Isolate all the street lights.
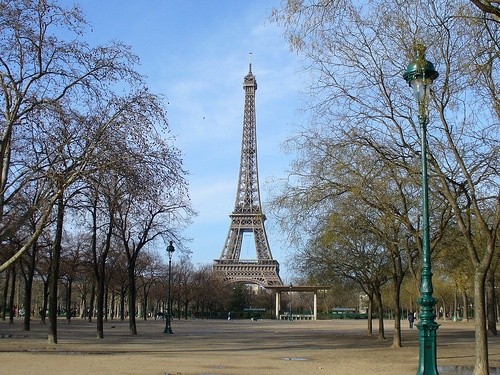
[289,284,293,321]
[163,241,175,334]
[402,43,442,375]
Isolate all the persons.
[408,311,414,328]
